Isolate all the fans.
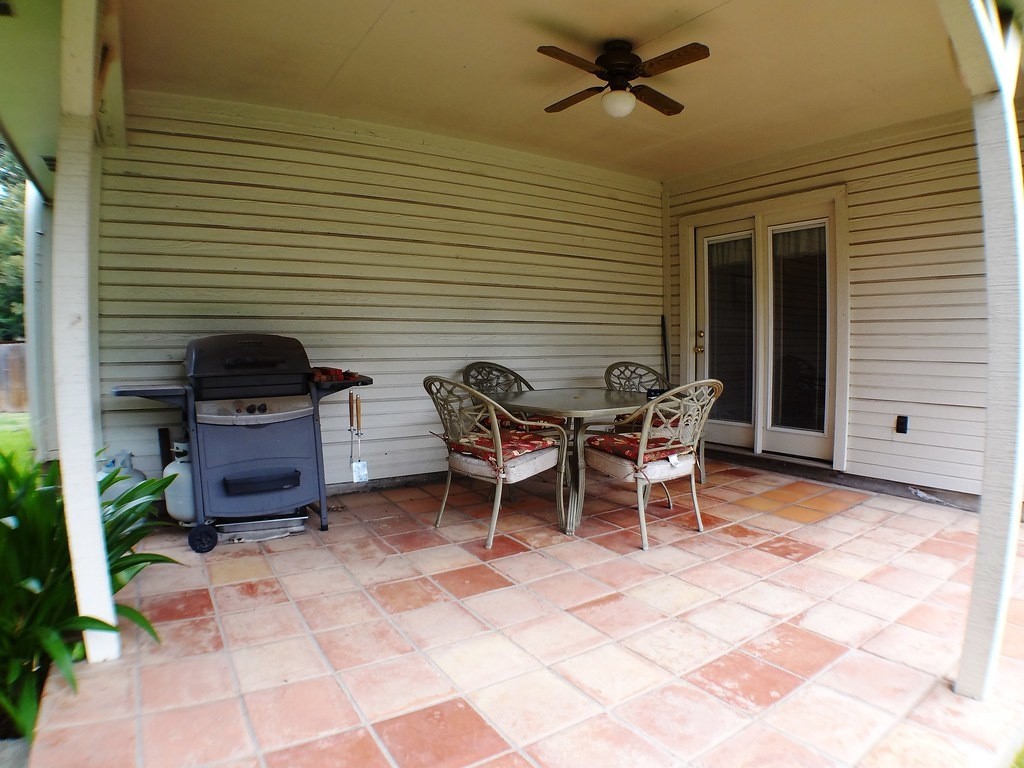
[536,39,710,117]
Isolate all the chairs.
[421,375,565,549]
[575,379,724,550]
[462,362,572,497]
[603,361,708,486]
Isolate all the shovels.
[352,394,368,483]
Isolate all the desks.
[476,387,688,536]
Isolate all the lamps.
[601,80,636,118]
